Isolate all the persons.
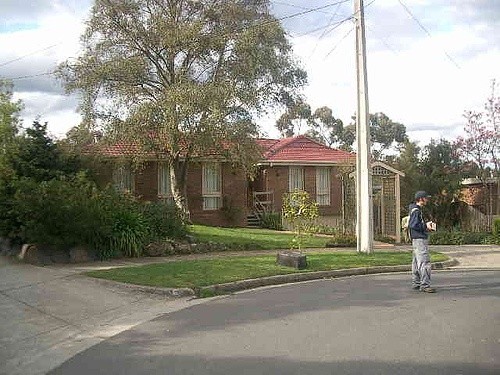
[408,191,436,293]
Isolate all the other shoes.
[421,287,436,292]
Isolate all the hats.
[414,191,431,200]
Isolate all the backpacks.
[402,208,423,243]
